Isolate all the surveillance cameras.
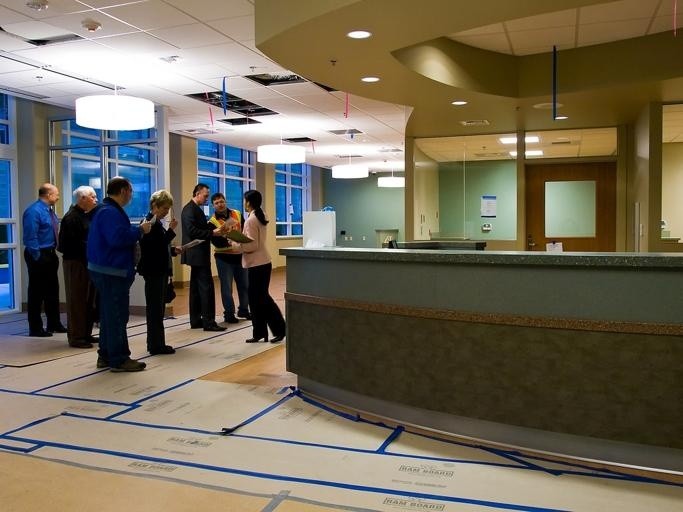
[27,0,46,11]
[82,22,99,30]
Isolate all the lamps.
[331,155,369,179]
[75,86,155,131]
[378,169,405,188]
[258,136,306,164]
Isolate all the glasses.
[166,206,176,223]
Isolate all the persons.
[241,190,287,343]
[88,177,152,373]
[137,190,184,356]
[22,182,67,336]
[208,192,251,324]
[58,186,99,351]
[181,182,233,332]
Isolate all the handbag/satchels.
[166,274,176,304]
[302,211,336,249]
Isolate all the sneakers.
[96,354,107,369]
[110,358,146,373]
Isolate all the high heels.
[270,332,287,343]
[247,333,269,343]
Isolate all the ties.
[49,206,60,250]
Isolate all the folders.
[227,230,254,243]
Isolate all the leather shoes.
[47,323,69,334]
[236,308,253,321]
[86,335,101,343]
[203,325,228,332]
[146,346,169,351]
[191,323,203,328]
[28,329,53,338]
[69,340,93,350]
[150,347,176,355]
[224,316,239,324]
